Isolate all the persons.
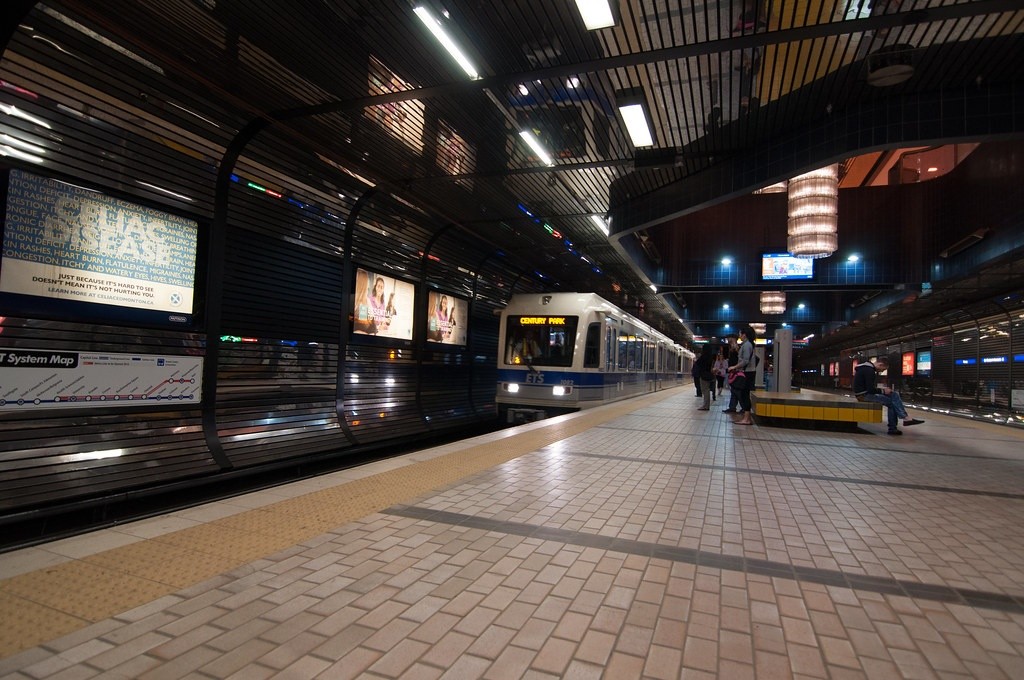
[429,291,456,342]
[691,325,755,424]
[793,369,801,386]
[853,357,925,435]
[355,270,396,334]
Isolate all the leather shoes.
[901,417,925,427]
[887,429,903,436]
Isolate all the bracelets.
[735,366,737,370]
[883,389,885,395]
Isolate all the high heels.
[718,390,722,396]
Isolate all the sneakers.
[722,408,737,414]
[735,408,746,414]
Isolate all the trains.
[491,292,697,426]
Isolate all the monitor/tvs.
[759,252,817,281]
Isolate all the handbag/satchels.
[728,369,747,390]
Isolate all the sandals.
[733,421,751,425]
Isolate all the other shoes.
[697,407,710,411]
[712,398,716,401]
[694,395,702,397]
